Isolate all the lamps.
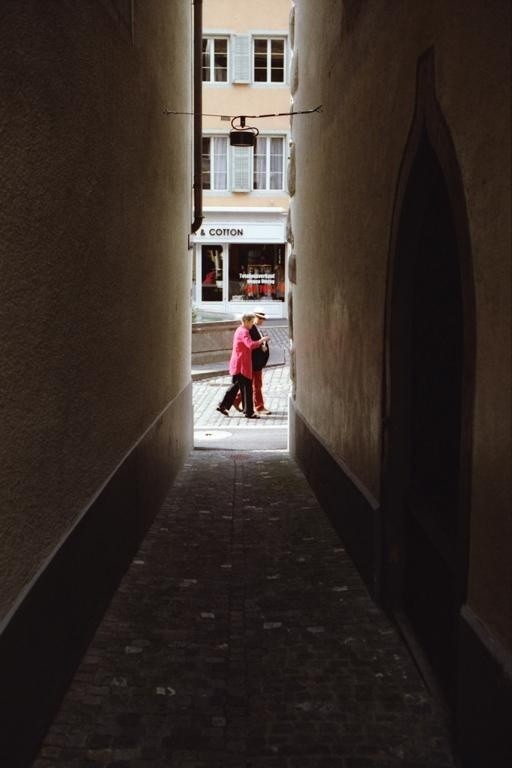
[229,117,259,147]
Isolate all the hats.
[253,310,266,319]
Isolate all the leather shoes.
[232,401,242,412]
[254,407,271,414]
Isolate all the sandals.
[216,405,228,416]
[245,413,260,419]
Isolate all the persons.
[216,313,271,419]
[233,312,272,414]
